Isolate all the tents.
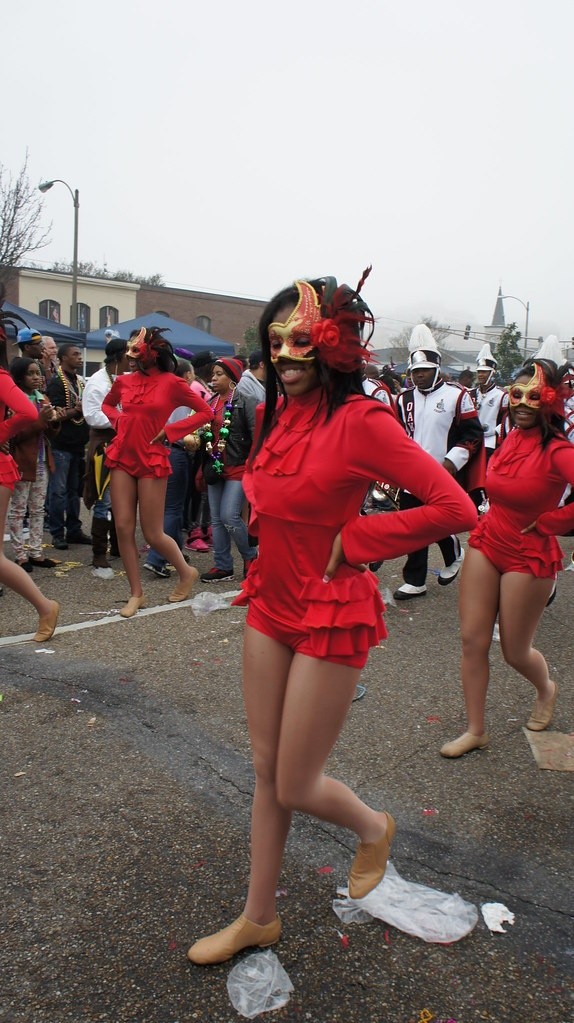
[1,301,87,379]
[85,313,235,357]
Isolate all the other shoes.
[169,567,200,602]
[120,591,145,617]
[33,600,59,642]
[28,556,56,568]
[347,811,395,899]
[369,560,384,572]
[166,553,190,566]
[438,548,465,586]
[16,560,33,572]
[91,566,114,580]
[526,680,558,731]
[109,547,121,557]
[142,562,171,576]
[439,732,490,757]
[393,583,428,601]
[188,911,281,963]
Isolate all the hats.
[475,344,498,374]
[11,328,42,346]
[405,323,442,373]
[534,336,567,370]
[214,357,243,383]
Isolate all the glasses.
[29,341,44,347]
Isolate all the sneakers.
[185,527,210,552]
[243,548,259,578]
[65,528,93,545]
[201,566,235,583]
[205,526,215,547]
[52,535,68,551]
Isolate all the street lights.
[497,296,529,358]
[38,180,79,329]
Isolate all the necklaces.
[57,366,84,426]
[204,387,236,474]
[106,366,122,411]
[195,376,213,394]
[36,363,41,392]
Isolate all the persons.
[7,357,62,573]
[142,347,267,582]
[393,367,486,600]
[82,339,142,569]
[439,357,574,758]
[46,343,93,550]
[360,364,414,572]
[12,328,67,530]
[458,359,574,515]
[0,326,59,642]
[187,277,479,963]
[101,330,214,617]
